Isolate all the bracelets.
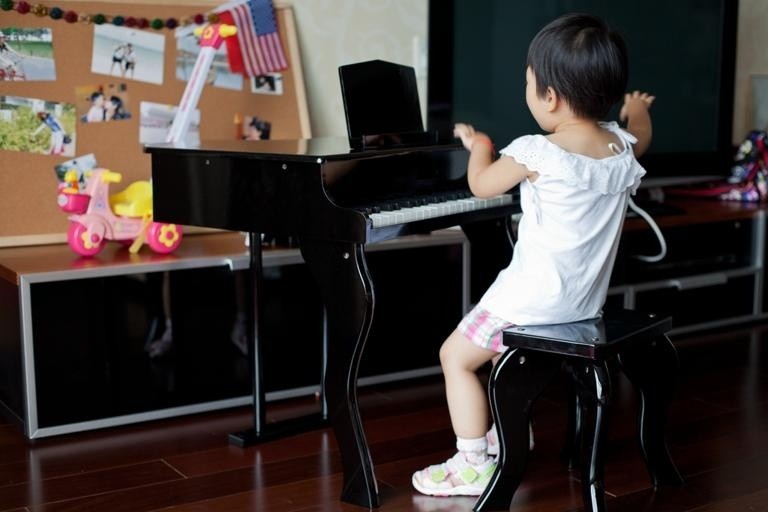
[473,137,497,158]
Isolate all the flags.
[215,0,288,77]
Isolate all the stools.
[473,306,709,511]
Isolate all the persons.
[0,32,24,81]
[32,109,67,155]
[258,75,271,90]
[104,96,122,121]
[245,120,266,140]
[86,92,104,122]
[108,42,136,81]
[412,6,658,498]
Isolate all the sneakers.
[412,453,497,497]
[487,422,535,456]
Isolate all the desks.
[1,181,766,441]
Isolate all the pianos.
[143,59,523,508]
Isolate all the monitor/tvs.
[426,0,740,176]
